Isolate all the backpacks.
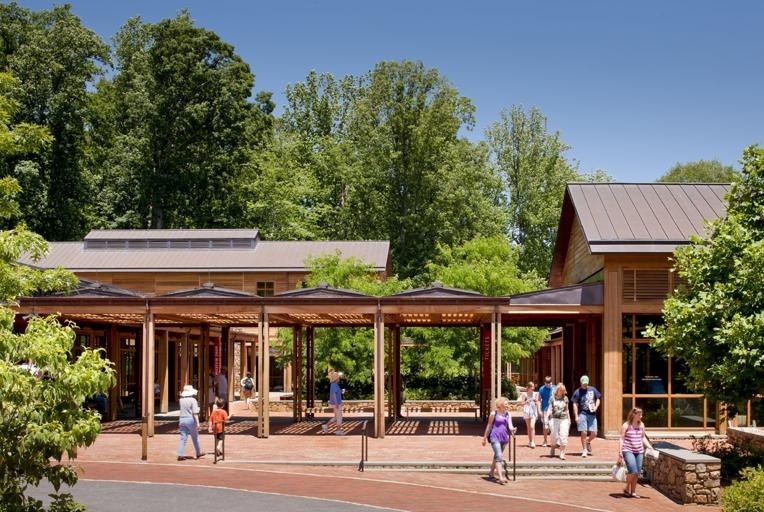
[245,378,253,390]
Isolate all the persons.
[321,371,346,436]
[482,397,517,483]
[214,366,227,408]
[205,371,216,415]
[240,372,254,411]
[207,398,235,456]
[538,374,558,448]
[617,407,656,497]
[176,384,205,461]
[547,384,570,460]
[518,381,543,447]
[571,376,601,458]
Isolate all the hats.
[329,372,339,382]
[580,376,589,385]
[180,386,197,396]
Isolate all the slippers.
[624,489,640,499]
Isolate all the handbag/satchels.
[612,465,626,481]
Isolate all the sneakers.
[528,441,592,460]
